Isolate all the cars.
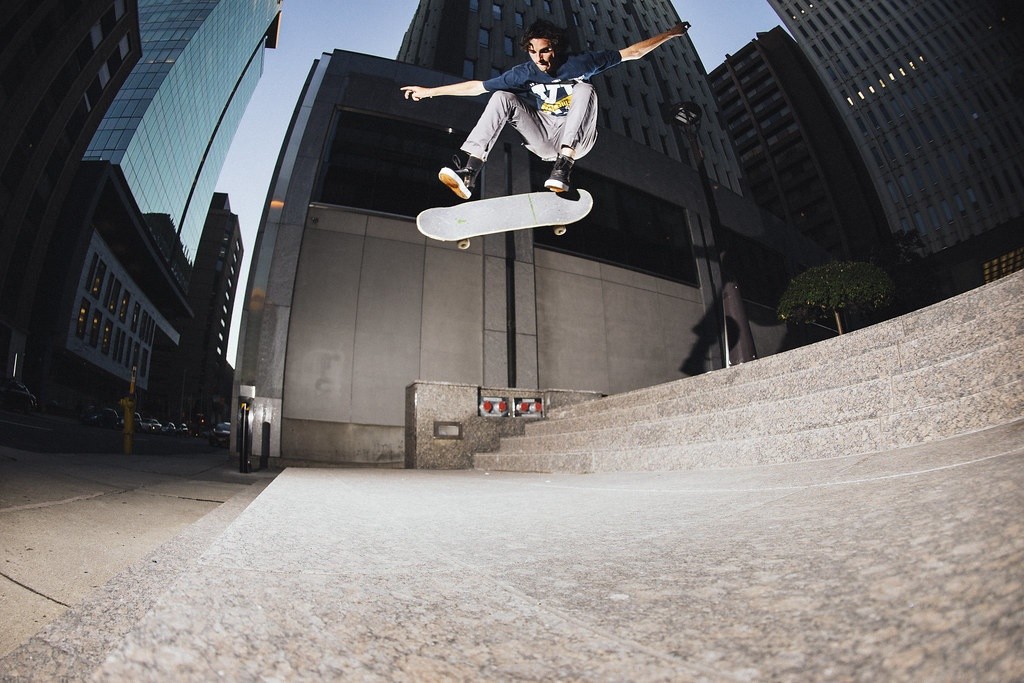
[81,406,188,437]
[0,379,37,416]
[209,421,231,448]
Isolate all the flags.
[265,12,280,49]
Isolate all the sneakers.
[545,163,575,198]
[439,167,479,199]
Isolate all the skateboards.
[416,187,595,250]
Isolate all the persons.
[398,19,693,200]
